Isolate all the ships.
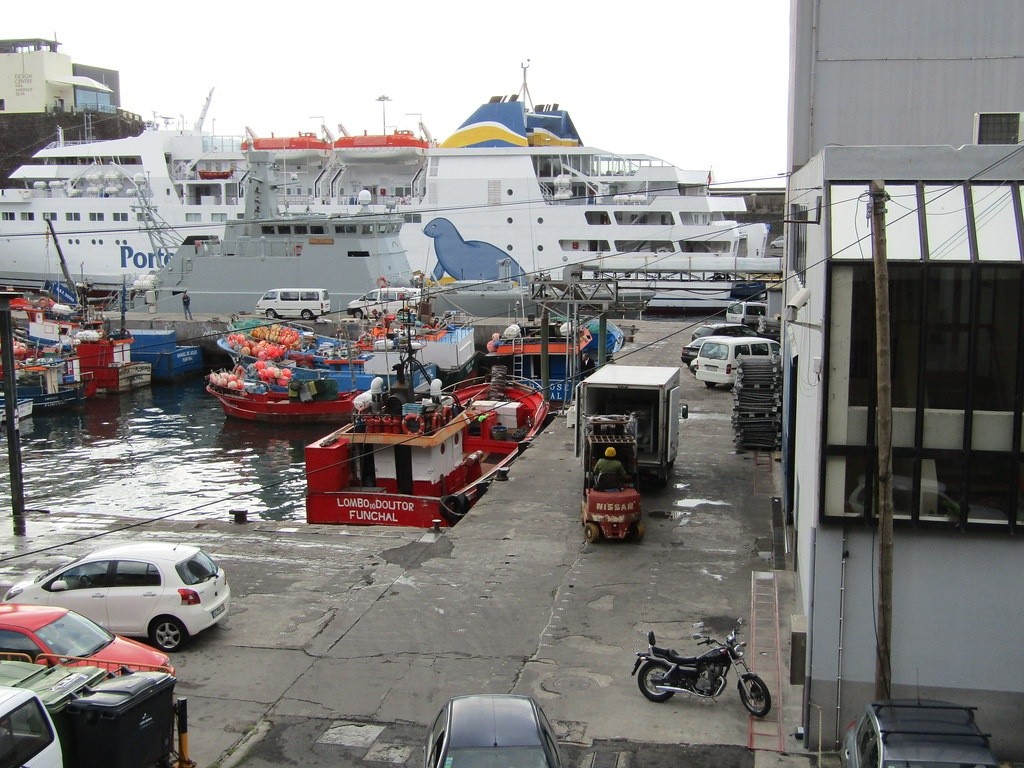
[0,40,787,307]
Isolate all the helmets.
[605,447,616,456]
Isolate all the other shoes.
[190,317,193,320]
[185,317,188,320]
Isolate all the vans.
[680,300,781,388]
[347,288,422,320]
[0,686,63,768]
[255,288,331,320]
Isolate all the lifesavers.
[421,324,433,329]
[293,245,303,256]
[377,276,387,288]
[442,406,453,426]
[38,297,48,308]
[195,240,201,248]
[431,412,441,433]
[402,414,425,435]
[435,323,440,329]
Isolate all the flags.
[708,170,712,186]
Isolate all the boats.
[216,314,438,394]
[241,138,332,166]
[206,381,362,423]
[484,314,625,402]
[0,398,33,421]
[305,364,551,528]
[10,308,203,394]
[333,136,429,165]
[396,316,475,378]
[0,338,98,408]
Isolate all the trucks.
[567,363,688,485]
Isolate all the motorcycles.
[630,618,772,717]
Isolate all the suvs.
[840,697,1000,768]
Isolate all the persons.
[182,291,193,321]
[107,327,135,343]
[593,446,632,491]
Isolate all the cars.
[422,693,562,768]
[0,603,176,681]
[1,542,231,653]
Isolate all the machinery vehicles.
[581,412,646,544]
